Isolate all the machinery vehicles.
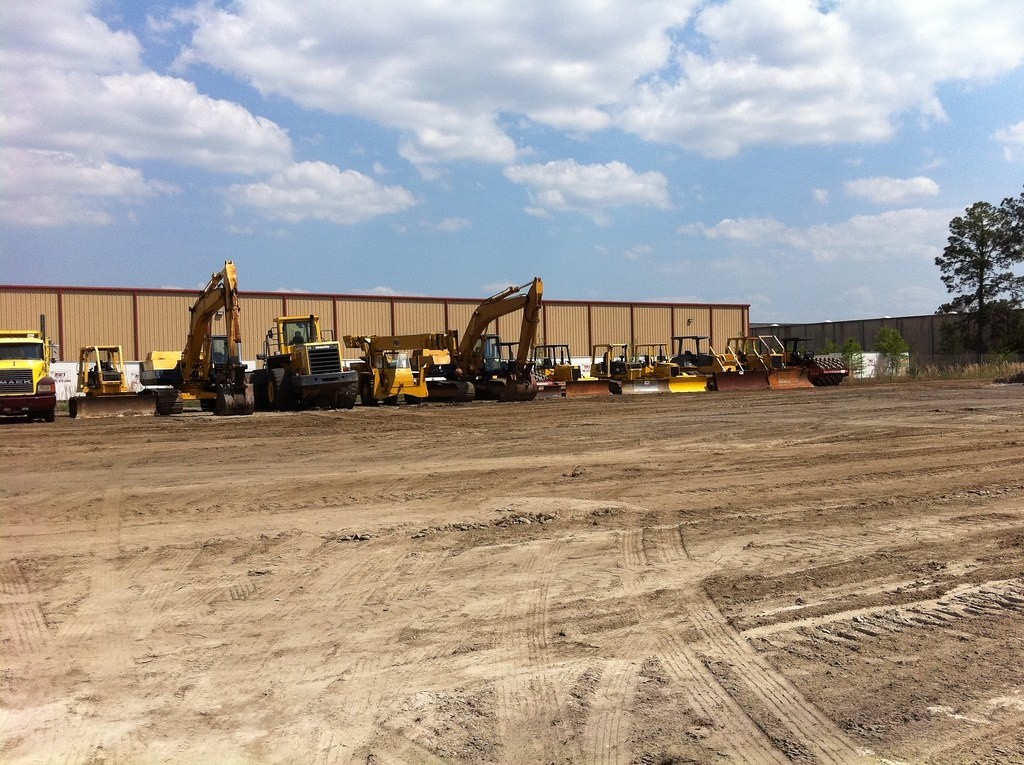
[249,313,358,411]
[0,330,58,423]
[66,345,156,419]
[137,260,254,416]
[468,330,851,398]
[409,276,545,402]
[342,328,463,407]
